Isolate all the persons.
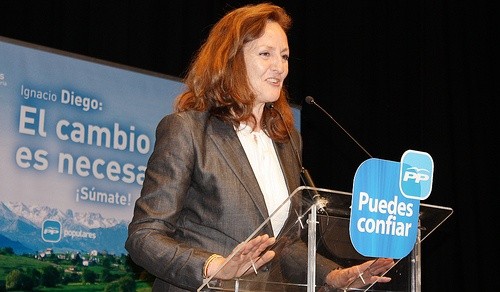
[124,3,395,292]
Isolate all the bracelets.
[332,267,342,271]
[202,254,223,278]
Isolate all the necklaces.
[244,122,260,144]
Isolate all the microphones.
[265,102,327,213]
[305,96,373,158]
